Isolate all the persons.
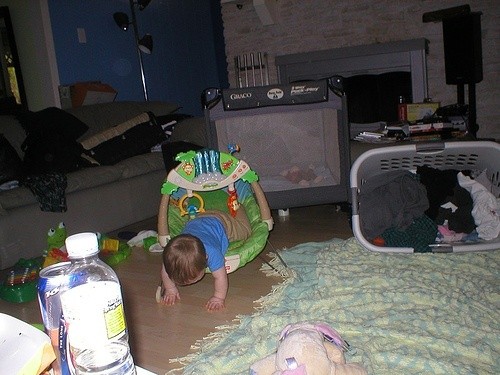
[160,202,253,311]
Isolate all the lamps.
[113,0,153,103]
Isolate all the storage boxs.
[407,103,440,122]
[350,140,500,253]
[72,84,117,108]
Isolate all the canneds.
[36,262,81,375]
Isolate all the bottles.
[60,232,137,375]
[398,96,407,122]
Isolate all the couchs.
[0,102,218,271]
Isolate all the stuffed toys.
[249,320,368,375]
